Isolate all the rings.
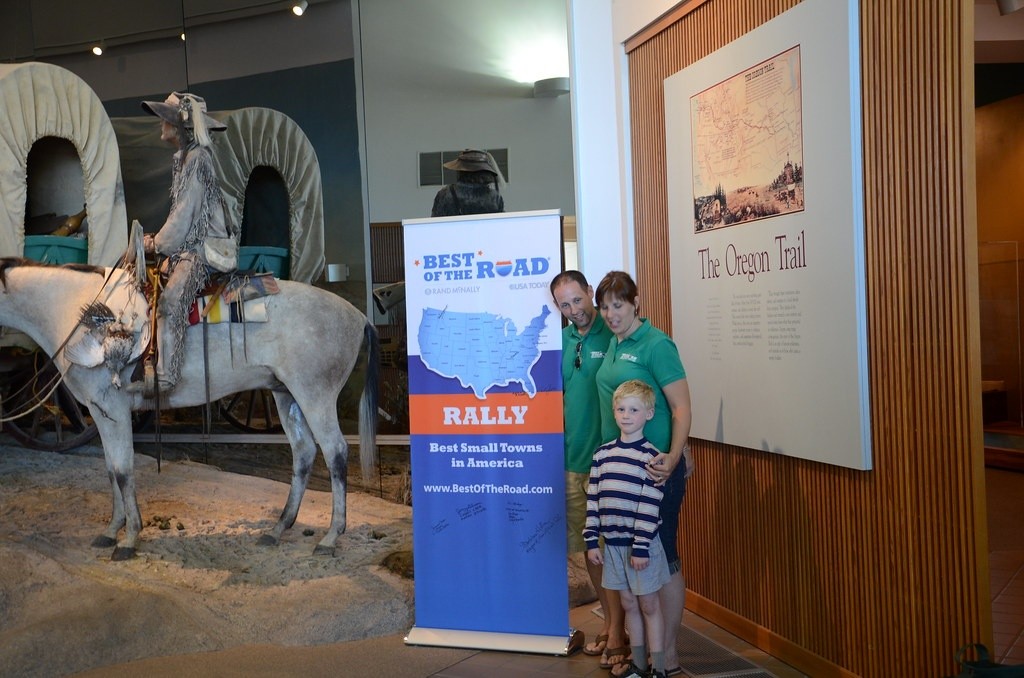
[658,476,663,482]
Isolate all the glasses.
[575,341,582,371]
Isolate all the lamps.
[93,38,107,55]
[293,0,308,16]
[532,77,569,99]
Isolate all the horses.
[0,258,379,563]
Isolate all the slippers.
[599,646,627,668]
[609,658,635,678]
[583,634,630,655]
[649,664,681,676]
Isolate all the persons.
[583,379,671,678]
[550,270,631,669]
[134,92,237,397]
[431,150,505,218]
[598,270,695,678]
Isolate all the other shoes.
[650,669,665,678]
[622,664,649,678]
